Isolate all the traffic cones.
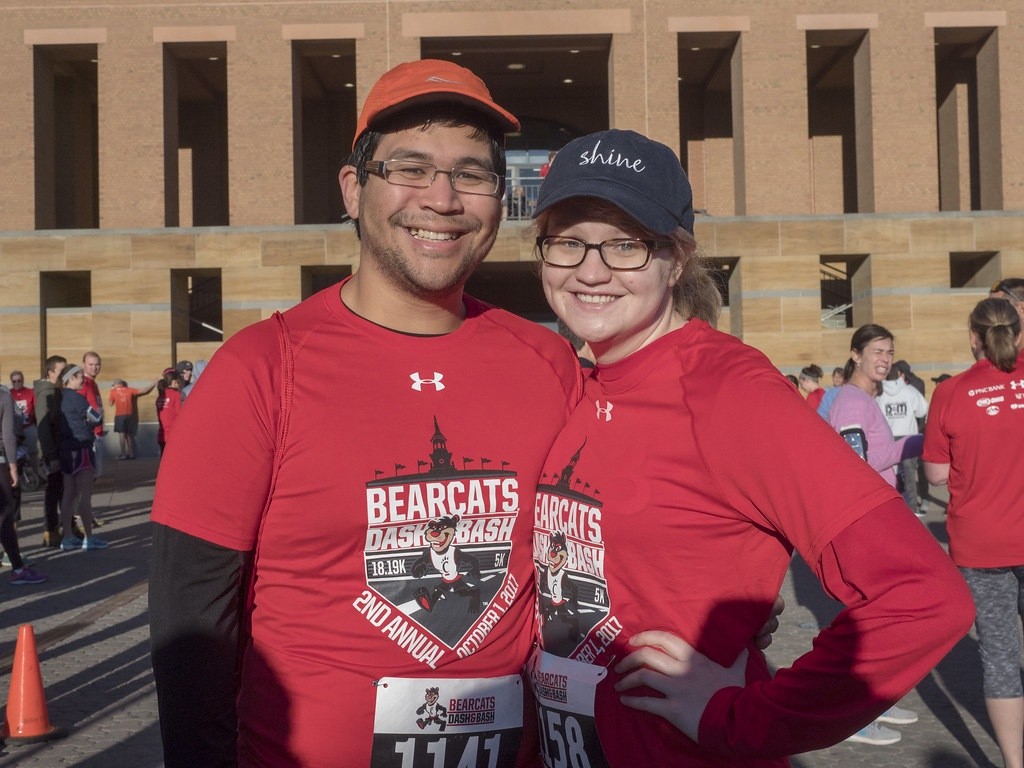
[0,625,69,747]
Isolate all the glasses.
[991,280,1023,303]
[362,159,505,195]
[12,379,21,384]
[534,234,675,270]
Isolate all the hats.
[112,379,124,386]
[932,373,951,382]
[533,129,695,237]
[351,60,520,160]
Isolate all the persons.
[828,321,927,743]
[522,122,977,768]
[0,351,110,588]
[156,359,212,462]
[107,379,158,460]
[141,57,799,768]
[985,276,1024,360]
[774,357,959,526]
[922,296,1024,767]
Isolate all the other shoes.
[44,527,62,545]
[912,508,927,518]
[69,525,84,539]
[115,454,136,461]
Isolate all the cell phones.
[88,407,102,422]
[841,428,867,463]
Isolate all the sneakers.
[848,724,901,745]
[82,534,109,550]
[61,536,84,550]
[876,706,920,725]
[10,564,47,586]
